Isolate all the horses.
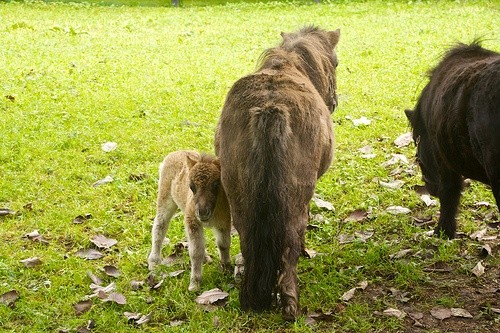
[214,26,341,319]
[148,150,233,293]
[404,31,500,239]
